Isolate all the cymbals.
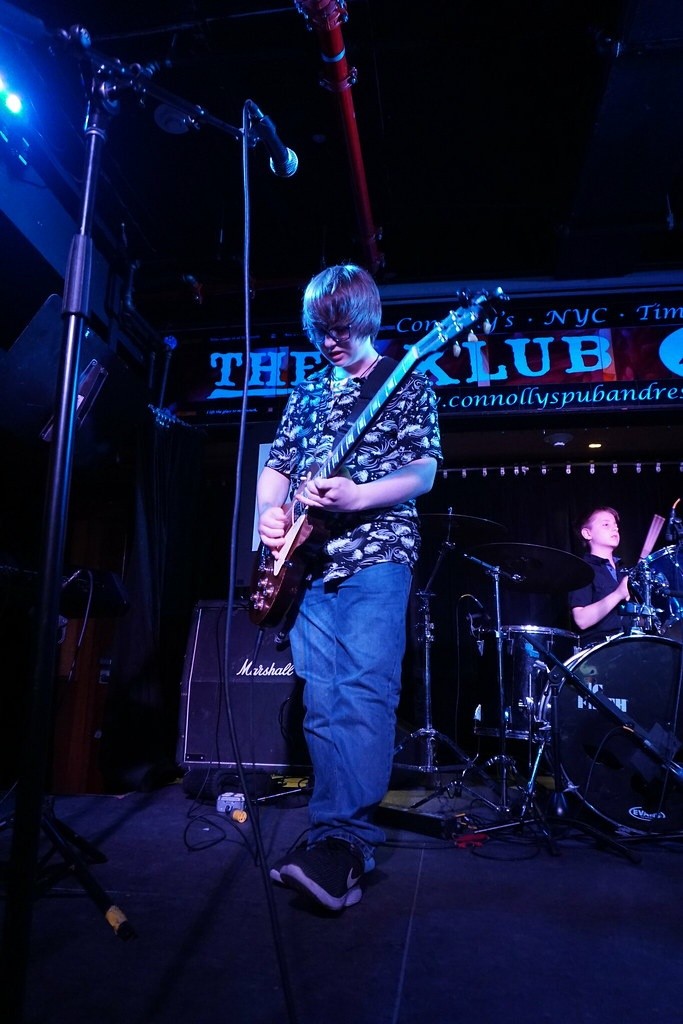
[470,541,596,593]
[415,512,508,544]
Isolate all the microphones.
[246,99,299,178]
[662,759,683,783]
[665,509,676,542]
[27,568,84,616]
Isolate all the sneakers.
[269,855,376,882]
[279,838,362,910]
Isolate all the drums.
[548,633,683,839]
[473,625,581,745]
[629,545,683,641]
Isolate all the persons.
[570,505,632,650]
[257,266,442,909]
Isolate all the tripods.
[0,604,135,939]
[393,524,643,866]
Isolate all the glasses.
[308,314,358,343]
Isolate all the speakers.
[175,600,314,778]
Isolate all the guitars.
[247,288,511,625]
